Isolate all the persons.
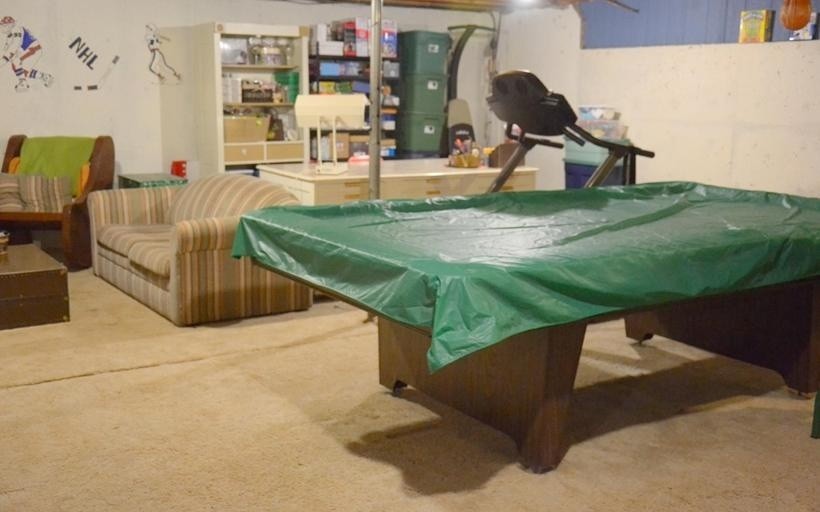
[0,16,52,90]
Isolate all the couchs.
[86,173,314,329]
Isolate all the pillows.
[1,172,74,214]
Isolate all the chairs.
[1,134,114,273]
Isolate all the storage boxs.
[561,104,629,163]
[396,31,453,154]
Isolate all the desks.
[118,172,189,189]
[232,181,820,474]
[258,153,541,207]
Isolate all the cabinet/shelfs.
[194,21,401,175]
[562,157,637,188]
